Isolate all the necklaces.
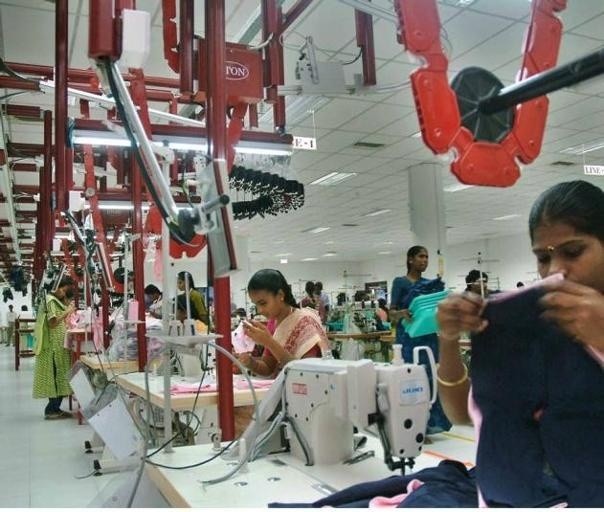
[289,305,294,313]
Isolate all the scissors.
[343,449,375,465]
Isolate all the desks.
[112,369,275,444]
[80,352,142,482]
[298,304,474,365]
[135,413,482,507]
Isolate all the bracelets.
[434,360,468,387]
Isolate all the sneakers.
[45,407,73,419]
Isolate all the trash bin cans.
[0,326,8,343]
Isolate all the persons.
[19,305,32,319]
[440,180,603,509]
[170,272,211,331]
[461,270,501,298]
[298,281,390,328]
[205,294,215,321]
[145,283,163,317]
[226,307,245,336]
[5,304,18,348]
[388,247,445,443]
[239,269,334,382]
[30,278,76,420]
